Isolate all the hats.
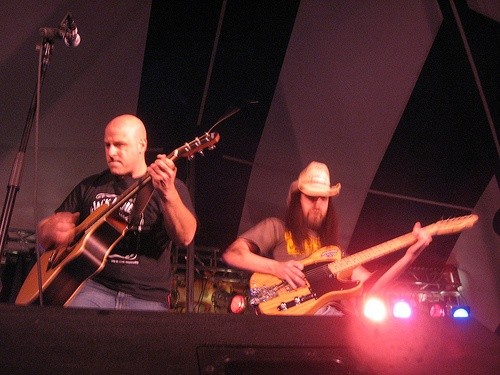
[286,161,341,205]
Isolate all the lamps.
[212,293,248,314]
[418,300,470,318]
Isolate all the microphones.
[64,15,80,48]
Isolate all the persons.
[38,115,198,311]
[222,162,431,318]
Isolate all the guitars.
[249,214,478,316]
[14,133,220,306]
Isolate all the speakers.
[0,305,500,375]
